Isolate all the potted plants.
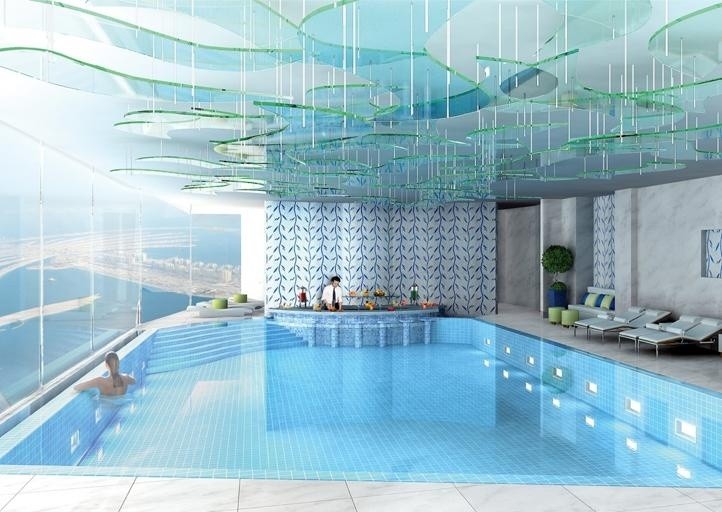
[542,245,574,307]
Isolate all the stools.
[562,310,579,328]
[235,293,248,303]
[548,308,563,323]
[211,297,229,310]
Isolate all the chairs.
[574,306,722,359]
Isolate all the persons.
[73,351,136,398]
[319,275,344,312]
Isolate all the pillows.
[583,291,599,305]
[595,294,615,309]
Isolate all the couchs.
[568,287,615,320]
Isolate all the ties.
[332,288,336,306]
